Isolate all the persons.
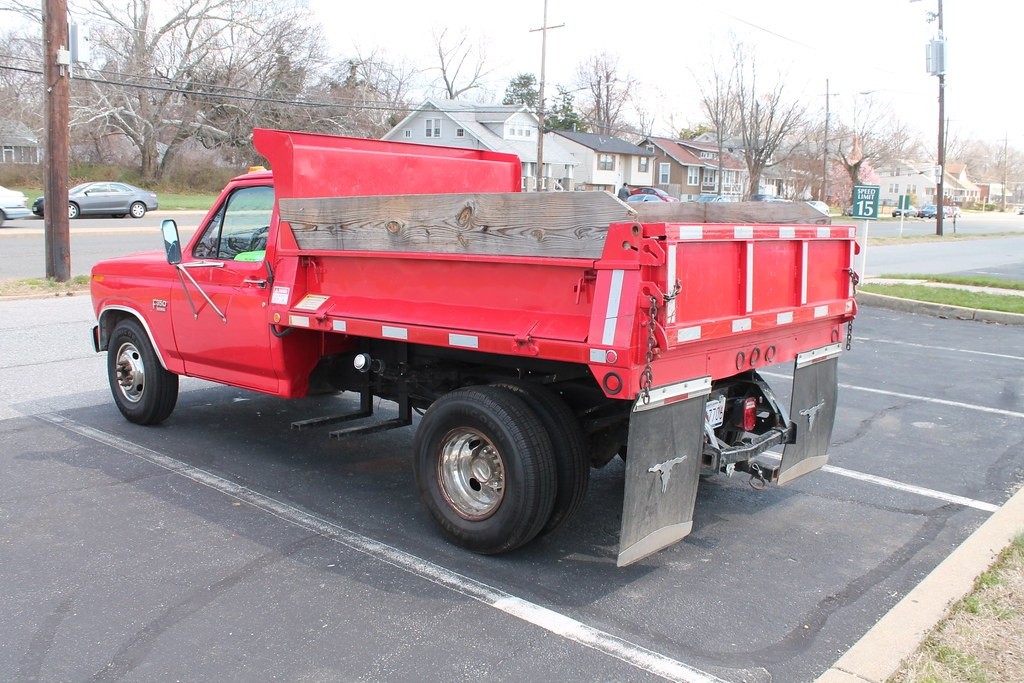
[618,183,631,200]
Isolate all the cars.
[696,193,725,203]
[951,205,961,218]
[892,208,903,217]
[626,194,666,203]
[747,194,777,204]
[32,181,160,220]
[808,200,829,216]
[917,204,945,220]
[904,205,918,217]
[629,186,679,203]
[0,185,32,226]
[943,205,952,218]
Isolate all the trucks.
[88,126,859,558]
